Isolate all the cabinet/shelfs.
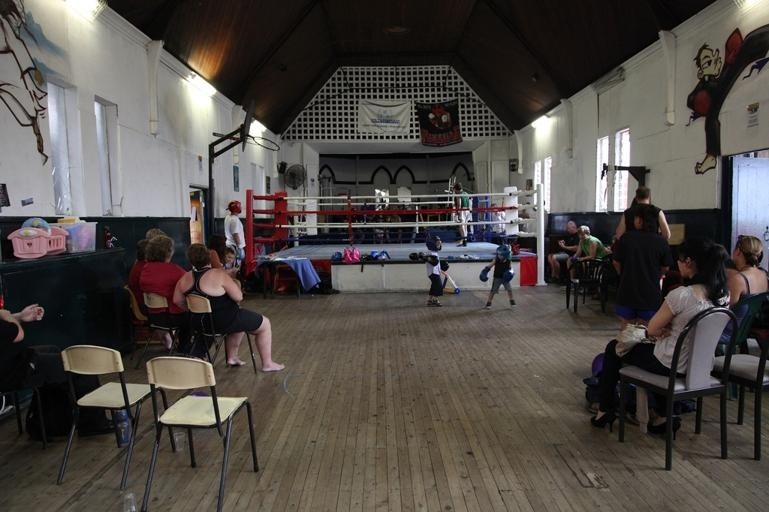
[0,247,129,403]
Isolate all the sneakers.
[433,298,443,307]
[486,301,491,309]
[510,300,517,308]
[424,299,437,307]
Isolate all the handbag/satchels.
[583,352,697,415]
[344,247,389,264]
[614,320,656,358]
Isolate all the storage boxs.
[50,222,98,253]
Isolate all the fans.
[284,164,307,190]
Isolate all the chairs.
[566,258,610,313]
[185,294,257,374]
[618,306,738,471]
[592,253,621,300]
[715,292,769,401]
[124,285,149,361]
[140,356,259,512]
[133,291,189,370]
[694,329,769,459]
[57,344,176,490]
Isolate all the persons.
[591,237,729,440]
[221,246,236,270]
[612,206,674,331]
[224,201,246,259]
[615,186,671,241]
[145,228,164,239]
[0,295,134,438]
[479,245,517,309]
[718,235,769,344]
[172,243,286,372]
[418,233,448,307]
[453,182,471,247]
[566,225,613,300]
[127,239,193,353]
[139,234,213,359]
[208,234,242,305]
[547,221,580,283]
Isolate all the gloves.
[427,255,439,266]
[409,253,419,260]
[440,260,449,271]
[480,267,490,282]
[503,270,514,282]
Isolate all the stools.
[0,384,49,449]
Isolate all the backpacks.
[25,383,74,440]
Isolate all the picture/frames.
[233,166,239,191]
[266,176,270,194]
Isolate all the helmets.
[426,236,441,251]
[495,245,511,264]
[226,201,241,213]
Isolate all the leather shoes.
[78,419,117,437]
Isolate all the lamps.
[509,158,518,172]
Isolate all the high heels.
[591,412,616,432]
[647,417,681,440]
